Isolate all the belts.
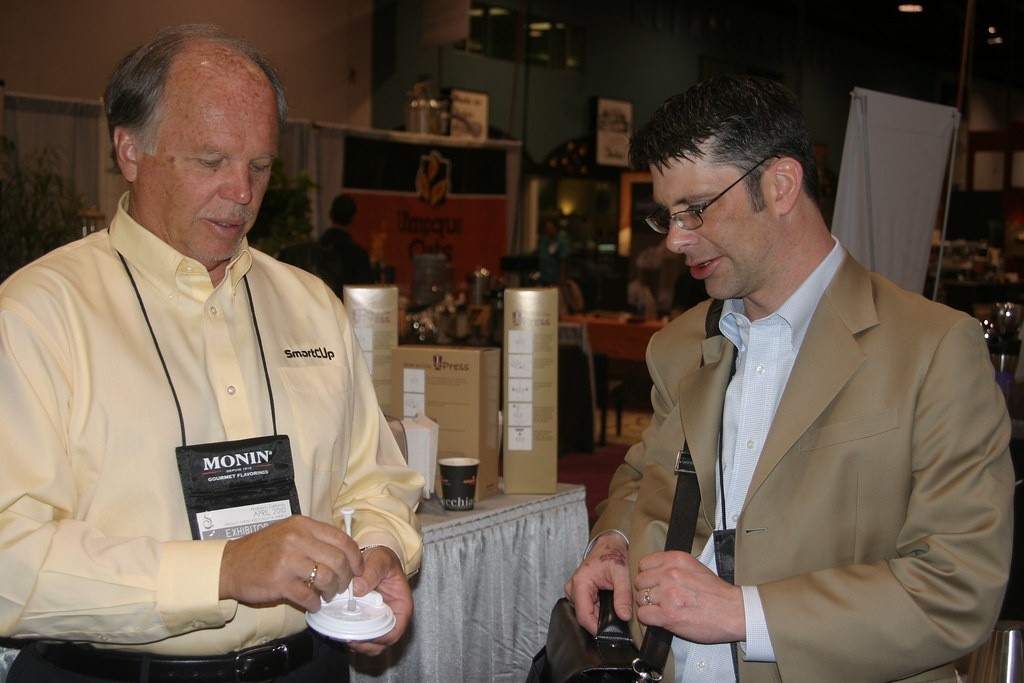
[28,627,329,682]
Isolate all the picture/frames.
[697,52,786,87]
[590,94,636,176]
[442,86,491,138]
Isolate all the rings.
[642,587,652,606]
[302,561,320,590]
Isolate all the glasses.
[642,154,781,234]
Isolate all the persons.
[537,251,588,318]
[401,236,447,315]
[302,189,383,303]
[626,259,665,314]
[562,70,1019,683]
[1,24,428,683]
[944,191,1003,245]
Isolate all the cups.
[438,455,481,511]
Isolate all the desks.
[349,473,590,683]
[559,308,671,447]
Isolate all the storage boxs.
[343,283,559,504]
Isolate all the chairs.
[557,322,626,448]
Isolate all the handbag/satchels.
[525,589,671,683]
